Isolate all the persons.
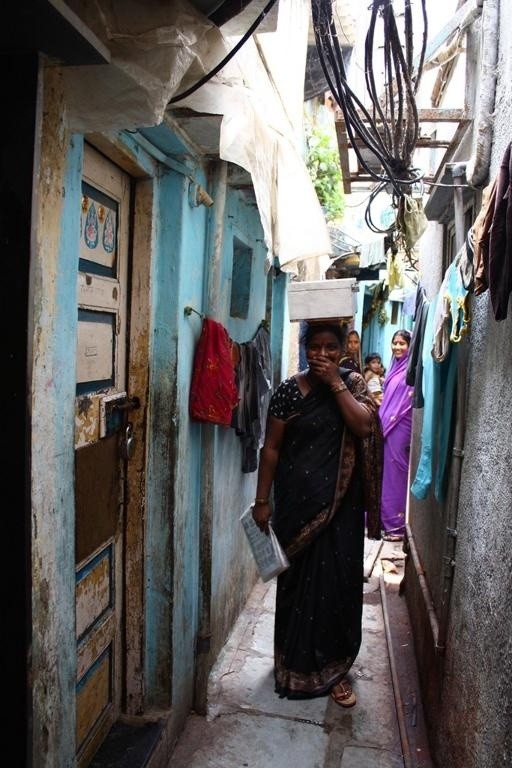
[364,353,384,407]
[379,328,415,541]
[252,323,385,707]
[338,330,362,374]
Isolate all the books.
[240,501,290,583]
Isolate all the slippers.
[330,679,356,707]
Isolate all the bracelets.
[255,498,268,504]
[331,379,348,395]
